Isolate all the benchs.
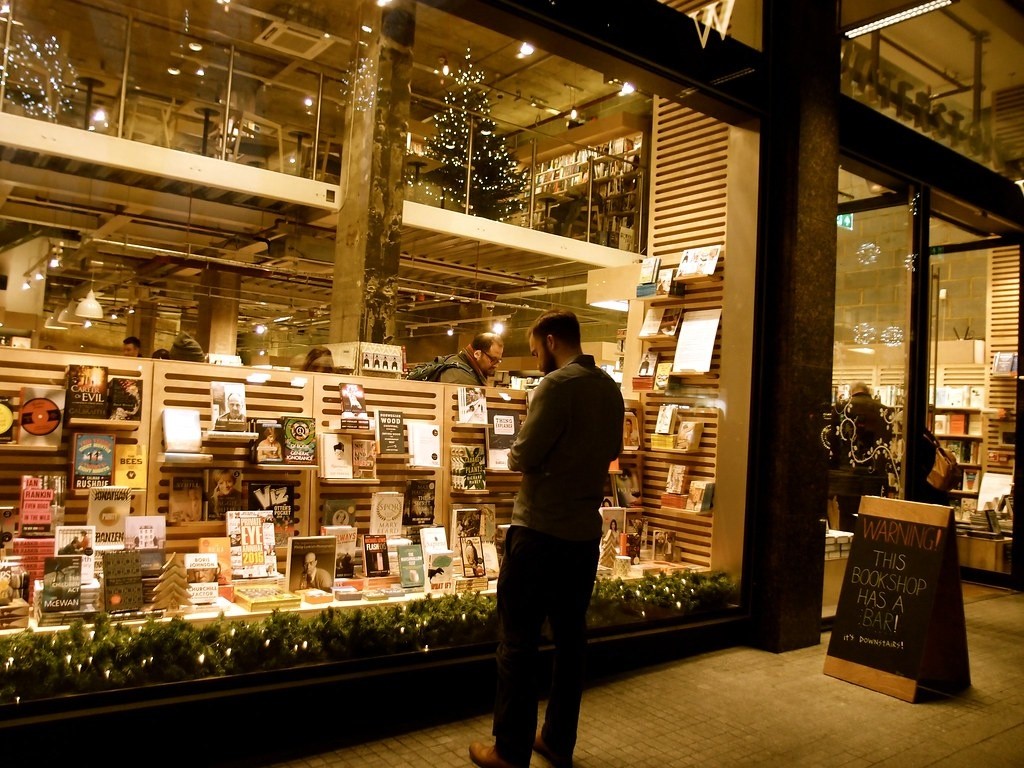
[534,181,602,240]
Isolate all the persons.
[299,552,332,591]
[435,331,504,386]
[624,418,640,447]
[257,427,284,464]
[829,381,891,533]
[617,462,641,506]
[122,336,143,358]
[186,568,217,583]
[216,392,246,429]
[468,308,624,768]
[152,349,169,360]
[301,345,333,374]
[207,470,241,519]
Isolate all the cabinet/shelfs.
[146,359,315,576]
[883,405,982,496]
[602,274,756,587]
[0,344,155,631]
[442,381,527,565]
[522,131,648,253]
[310,372,445,578]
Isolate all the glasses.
[482,350,503,365]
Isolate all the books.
[522,136,640,251]
[0,259,1019,613]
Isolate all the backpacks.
[405,354,478,382]
[923,431,963,491]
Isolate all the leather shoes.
[533,729,553,760]
[469,742,512,768]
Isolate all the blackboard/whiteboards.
[824,495,954,706]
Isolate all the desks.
[169,97,237,158]
[522,191,575,233]
[63,66,121,129]
[270,122,336,178]
[404,152,446,203]
